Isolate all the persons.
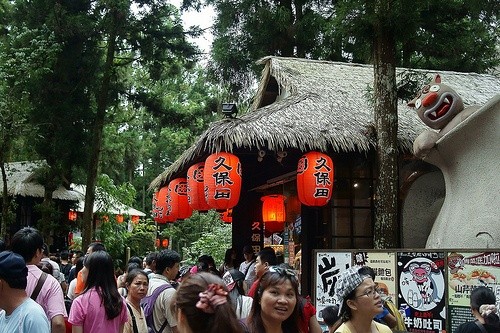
[116,249,241,333]
[65,242,131,333]
[452,288,500,333]
[321,265,408,333]
[0,227,73,333]
[220,246,323,333]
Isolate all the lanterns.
[168,178,192,219]
[186,162,211,213]
[163,239,168,247]
[157,186,176,223]
[262,197,286,233]
[156,239,160,246]
[103,215,108,222]
[152,192,167,224]
[132,216,139,224]
[116,214,123,223]
[204,151,242,209]
[223,209,232,224]
[69,211,77,220]
[296,150,333,206]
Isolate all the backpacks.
[140,284,173,333]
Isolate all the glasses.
[356,286,381,298]
[268,265,296,276]
[42,246,45,252]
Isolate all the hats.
[0,250,28,277]
[222,269,246,291]
[243,245,253,254]
[333,264,371,316]
